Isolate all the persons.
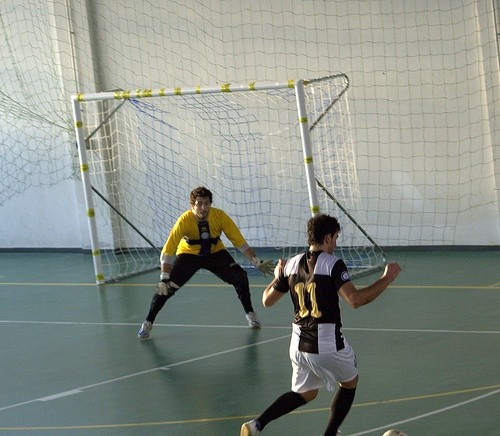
[138,186,276,339]
[238,212,405,435]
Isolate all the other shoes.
[240,419,259,436]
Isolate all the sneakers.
[245,312,261,327]
[138,320,153,340]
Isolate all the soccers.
[383,428,407,436]
